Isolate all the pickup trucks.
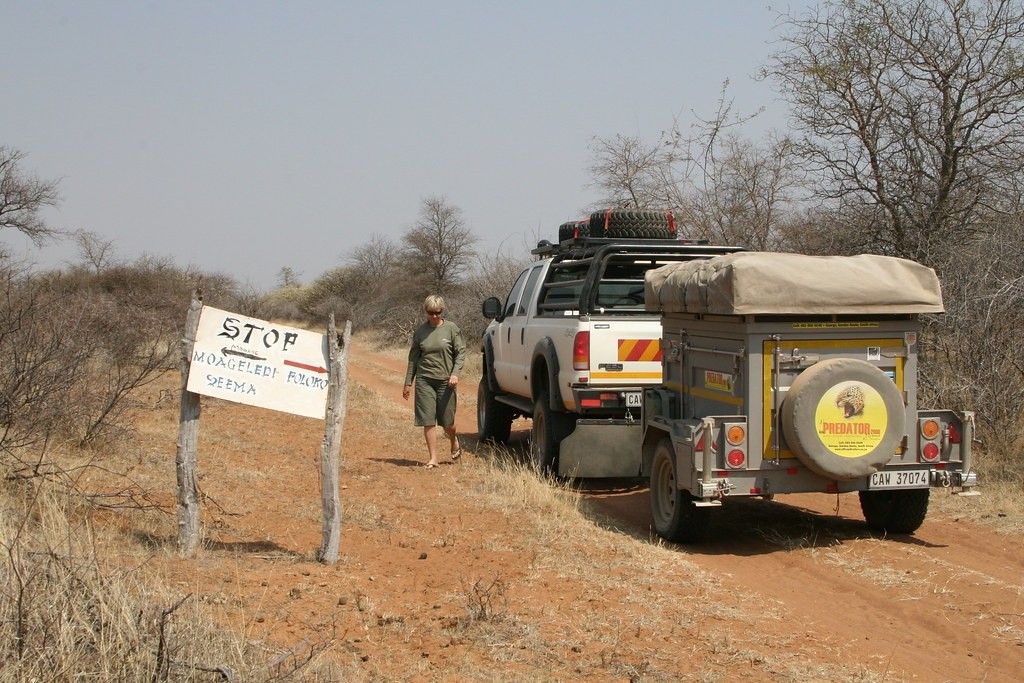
[477,208,756,484]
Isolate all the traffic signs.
[185,305,331,420]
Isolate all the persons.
[402,295,467,471]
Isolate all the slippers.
[422,463,439,470]
[450,435,461,460]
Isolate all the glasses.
[427,309,442,315]
